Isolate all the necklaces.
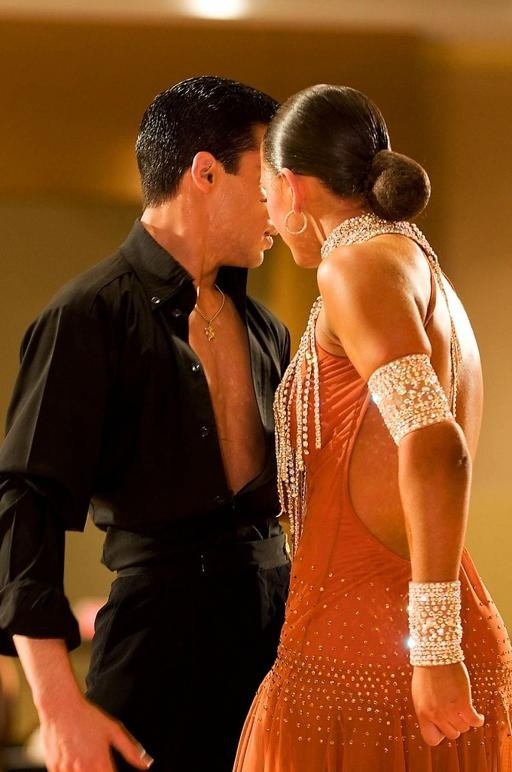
[318,212,394,258]
[195,282,227,342]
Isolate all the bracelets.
[406,580,465,670]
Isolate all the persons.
[0,73,292,772]
[229,82,512,771]
